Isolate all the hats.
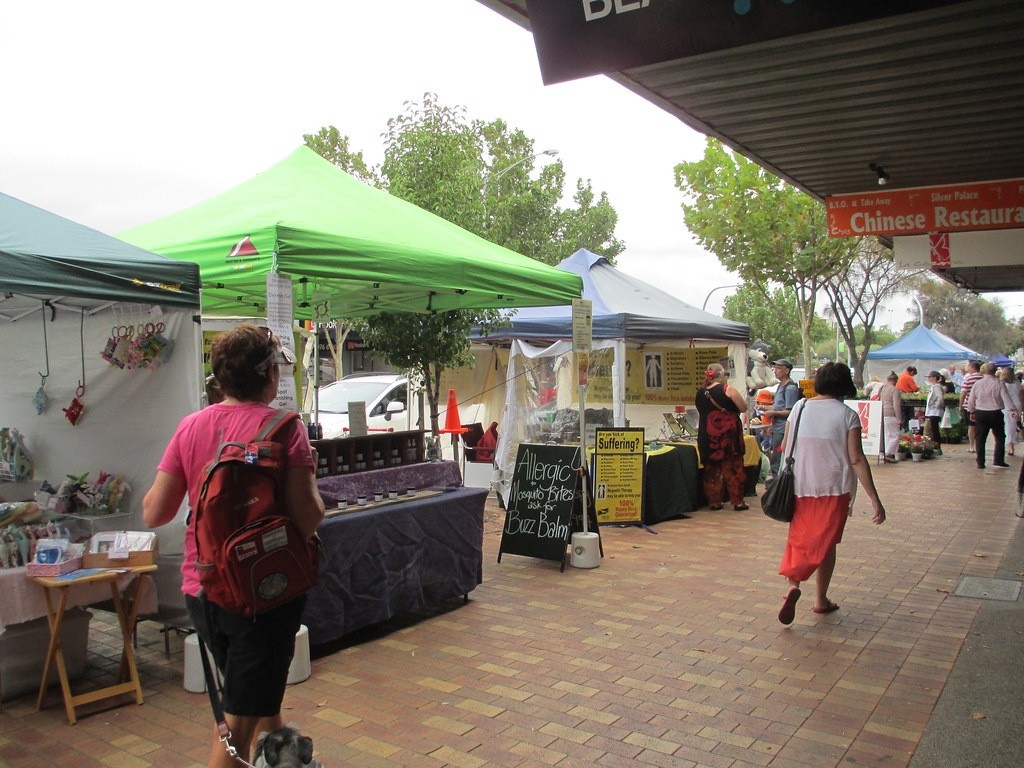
[771,359,793,370]
[254,335,297,374]
[755,390,773,403]
[924,371,941,377]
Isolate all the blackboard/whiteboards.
[501,443,600,560]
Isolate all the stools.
[461,422,496,484]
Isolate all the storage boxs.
[123,553,191,624]
[0,610,93,704]
[27,558,81,576]
[83,539,161,567]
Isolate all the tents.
[0,193,209,633]
[115,144,584,438]
[867,325,1015,395]
[408,246,751,442]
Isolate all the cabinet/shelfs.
[309,429,432,478]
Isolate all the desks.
[641,435,762,528]
[297,486,489,645]
[0,560,158,682]
[25,565,159,723]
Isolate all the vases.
[895,452,907,460]
[911,452,923,462]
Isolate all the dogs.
[251,724,324,768]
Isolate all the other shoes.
[1007,450,1014,455]
[885,456,898,463]
[966,449,975,453]
[734,502,749,510]
[993,461,1009,468]
[710,504,723,510]
[976,462,985,468]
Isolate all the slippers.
[814,599,839,613]
[779,588,801,624]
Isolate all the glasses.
[258,326,272,343]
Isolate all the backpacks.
[194,408,328,621]
[782,381,806,400]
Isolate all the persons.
[751,359,802,479]
[695,362,751,512]
[864,361,1024,469]
[779,363,886,625]
[142,324,325,768]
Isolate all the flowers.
[899,431,931,455]
[705,368,715,380]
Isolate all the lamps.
[875,166,891,185]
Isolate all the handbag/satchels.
[761,469,794,522]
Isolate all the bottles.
[357,495,367,506]
[307,422,324,441]
[314,438,417,478]
[374,492,384,502]
[389,490,398,500]
[407,487,416,496]
[338,499,348,509]
